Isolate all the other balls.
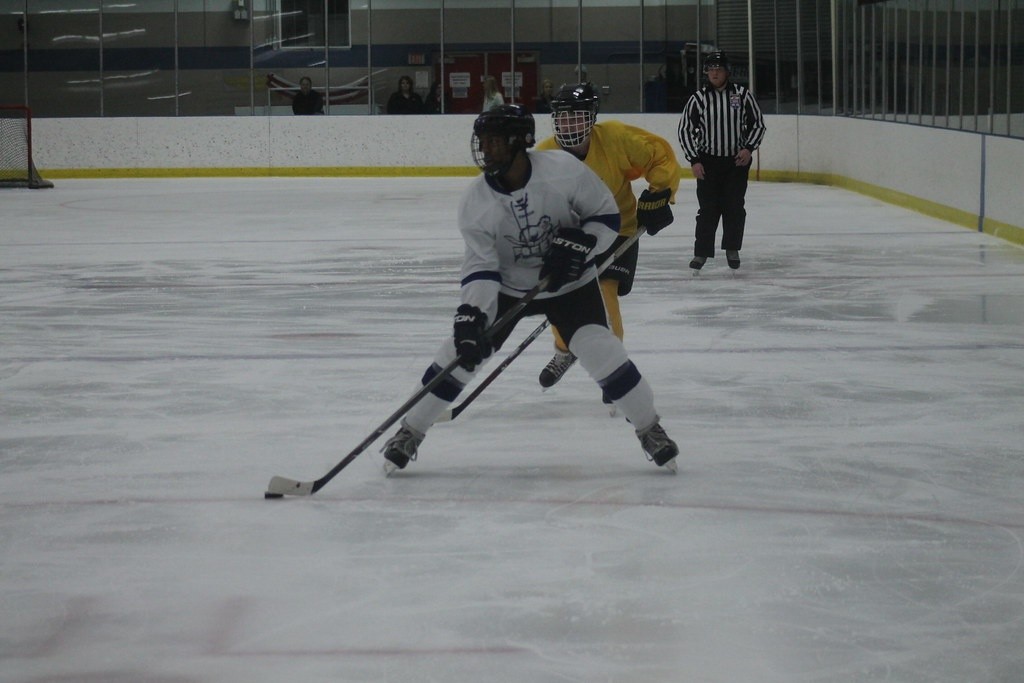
[263,492,284,501]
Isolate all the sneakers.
[379,416,426,477]
[726,250,740,275]
[538,341,577,392]
[603,389,616,418]
[689,255,706,277]
[625,414,679,473]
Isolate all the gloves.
[636,188,674,236]
[454,304,492,372]
[539,228,598,293]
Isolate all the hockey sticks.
[266,271,554,497]
[434,227,649,423]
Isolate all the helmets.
[552,81,602,147]
[471,102,536,179]
[703,51,731,75]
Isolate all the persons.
[482,76,504,112]
[384,83,681,469]
[678,54,766,270]
[292,77,326,115]
[574,66,601,107]
[386,75,430,114]
[533,79,554,114]
